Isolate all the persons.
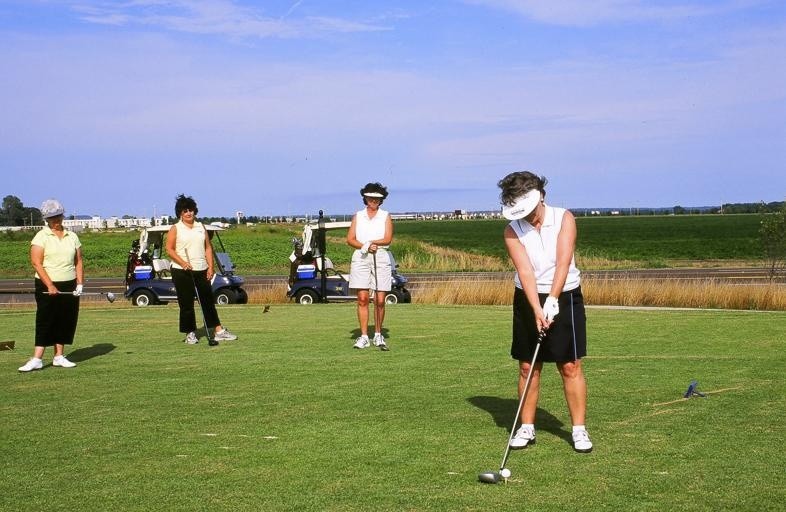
[347,181,393,350]
[18,198,84,372]
[497,171,594,453]
[166,193,239,345]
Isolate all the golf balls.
[498,467,513,480]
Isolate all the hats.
[40,200,65,219]
[364,191,383,199]
[502,189,539,221]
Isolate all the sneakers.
[509,429,536,450]
[373,336,386,347]
[354,338,369,348]
[18,358,43,371]
[52,355,76,368]
[185,332,198,344]
[214,329,237,341]
[572,429,592,453]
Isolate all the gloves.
[542,296,560,325]
[73,285,84,296]
[361,241,372,255]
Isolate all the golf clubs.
[42,290,115,303]
[370,250,388,350]
[478,317,551,482]
[183,248,218,346]
